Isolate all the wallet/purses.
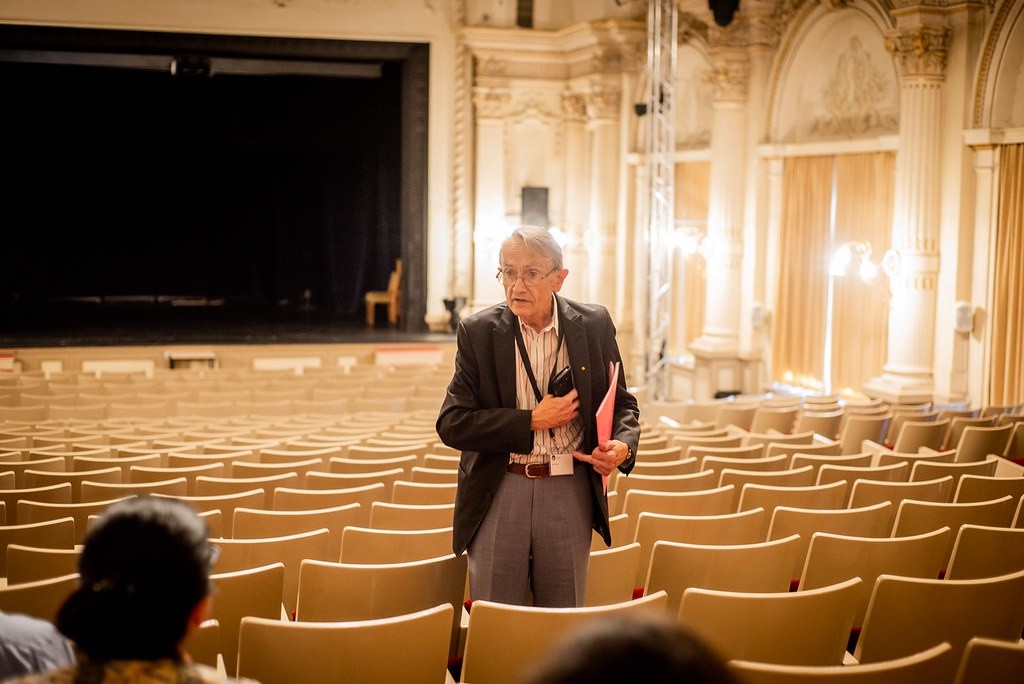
[552,366,574,398]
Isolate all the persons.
[3,499,262,684]
[0,610,77,684]
[523,617,743,684]
[435,225,641,608]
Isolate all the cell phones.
[552,368,574,397]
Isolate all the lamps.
[832,241,904,285]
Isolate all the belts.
[507,463,549,479]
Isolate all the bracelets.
[627,449,631,459]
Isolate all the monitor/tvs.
[522,188,548,226]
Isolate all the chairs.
[366,259,404,327]
[0,351,1024,684]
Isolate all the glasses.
[496,268,556,285]
